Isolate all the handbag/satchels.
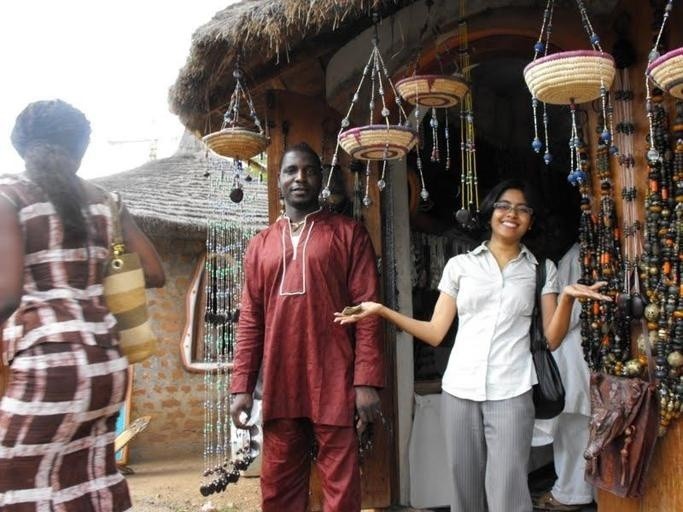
[526,256,566,424]
[99,190,161,365]
[584,318,660,498]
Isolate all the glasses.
[492,197,534,216]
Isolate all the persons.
[532,240,597,511]
[227,134,391,512]
[0,97,168,512]
[331,174,617,511]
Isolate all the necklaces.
[290,220,305,227]
